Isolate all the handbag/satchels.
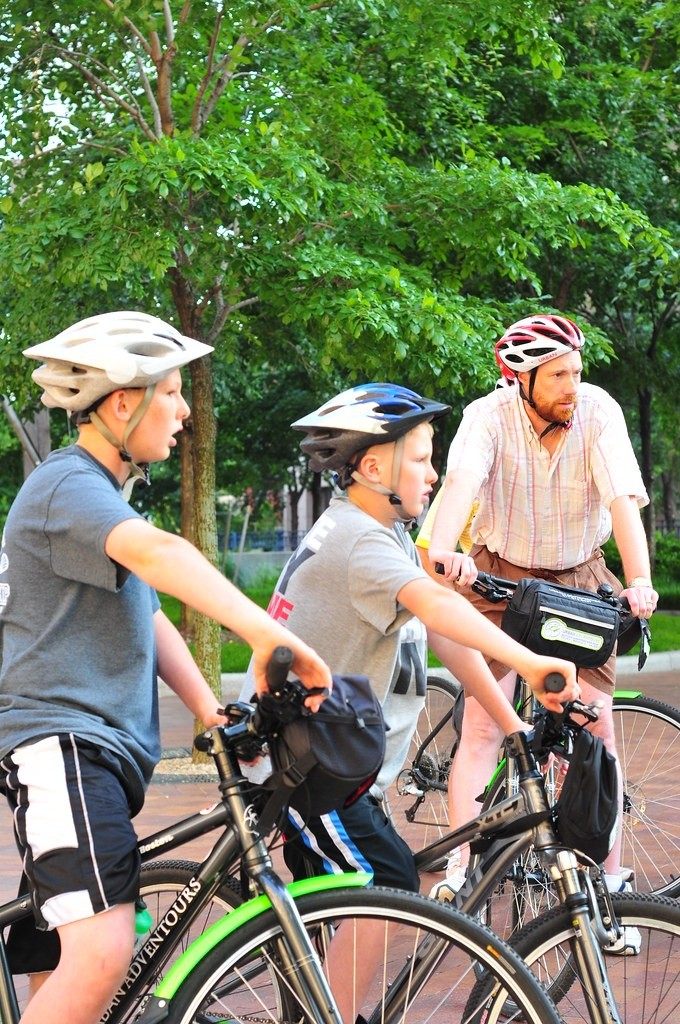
[269,673,391,818]
[533,718,618,868]
[499,578,619,669]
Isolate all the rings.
[646,601,654,604]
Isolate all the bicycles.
[0,564,680,1024]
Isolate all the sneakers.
[599,875,642,955]
[429,876,486,926]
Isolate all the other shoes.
[618,867,635,882]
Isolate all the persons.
[0,311,333,1024]
[235,382,582,1024]
[428,314,659,955]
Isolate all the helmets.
[21,312,216,411]
[289,383,452,472]
[495,376,520,390]
[495,314,585,380]
